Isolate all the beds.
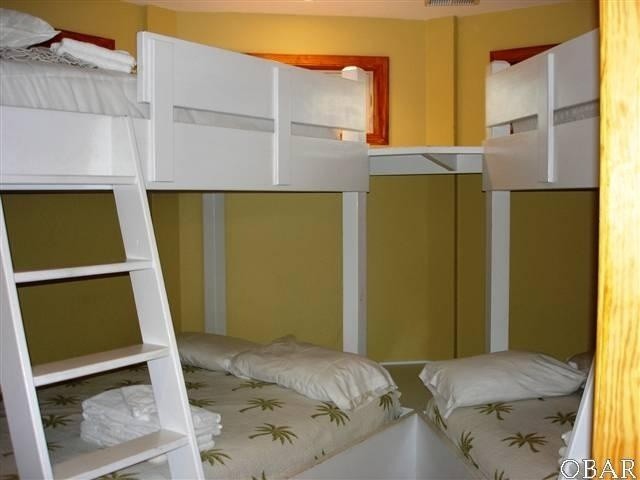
[0,38,404,480]
[423,14,604,480]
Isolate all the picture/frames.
[488,41,562,70]
[248,53,393,146]
[31,24,116,55]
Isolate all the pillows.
[0,5,62,52]
[416,348,590,416]
[233,328,399,411]
[175,333,263,373]
[564,349,594,394]
